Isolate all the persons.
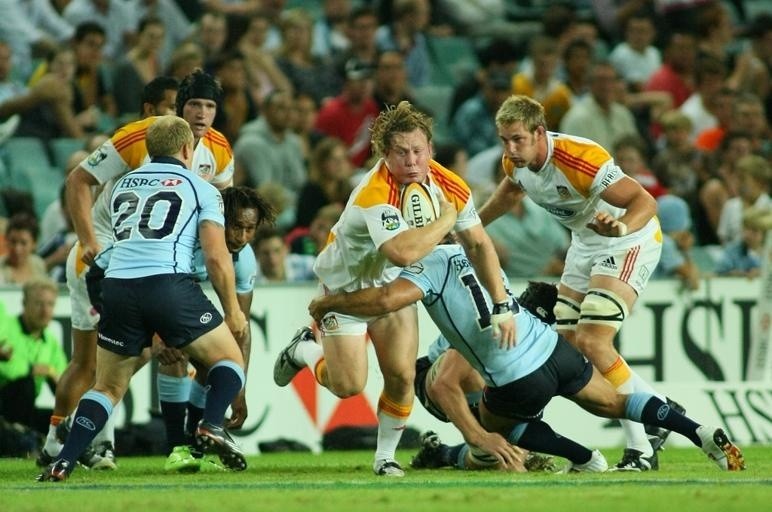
[408,280,557,469]
[273,99,516,476]
[156,183,286,472]
[449,93,686,475]
[36,115,249,482]
[309,231,746,475]
[31,65,237,471]
[2,2,772,290]
[1,282,68,459]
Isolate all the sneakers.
[163,419,247,473]
[274,326,317,387]
[36,419,117,481]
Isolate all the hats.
[657,195,691,232]
